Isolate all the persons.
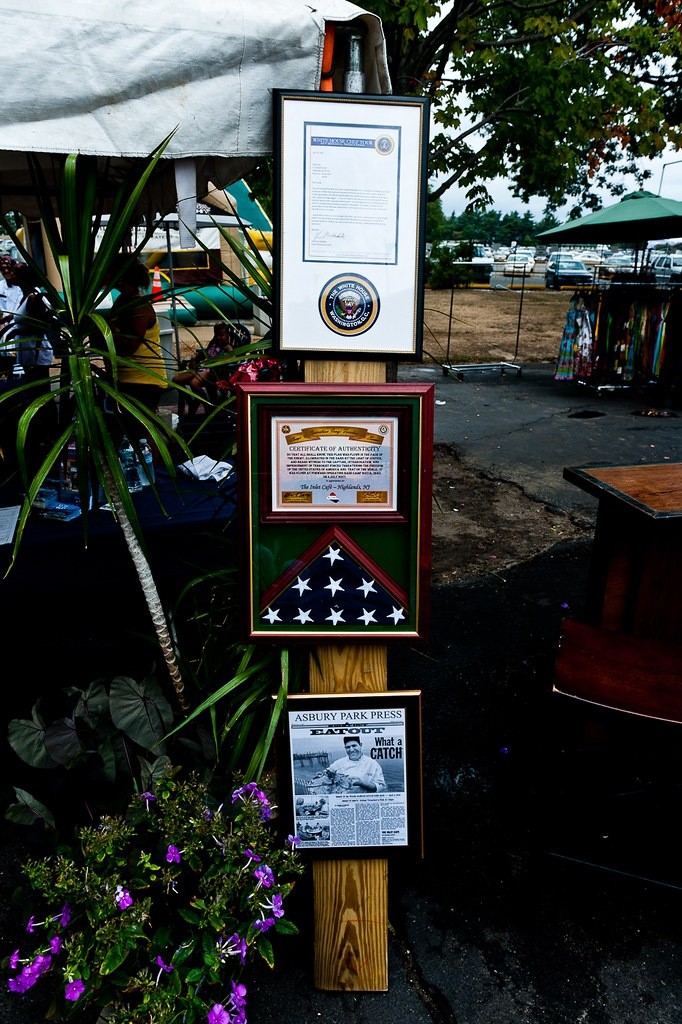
[103,253,167,439]
[0,254,52,447]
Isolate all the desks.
[548,457,682,722]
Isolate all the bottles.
[135,438,155,486]
[118,435,143,493]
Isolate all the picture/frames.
[231,381,436,644]
[270,690,425,861]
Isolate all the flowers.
[0,762,306,1024]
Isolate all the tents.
[534,188,682,291]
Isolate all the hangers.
[569,282,676,303]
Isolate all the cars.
[545,259,594,291]
[599,257,636,278]
[454,246,682,275]
[648,253,682,283]
[503,253,533,278]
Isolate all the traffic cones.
[151,266,168,302]
